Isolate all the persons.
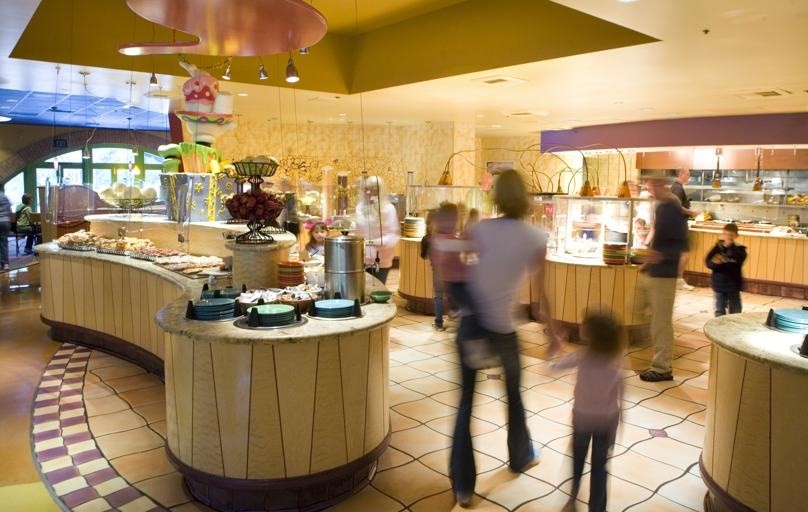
[419,169,553,511]
[16,192,34,254]
[0,191,12,270]
[300,222,329,262]
[624,170,700,291]
[542,308,626,511]
[705,224,747,316]
[633,170,683,382]
[347,175,402,284]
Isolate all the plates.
[277,262,305,287]
[201,288,244,299]
[771,308,808,332]
[313,299,356,317]
[247,304,296,326]
[631,248,650,265]
[793,226,808,233]
[602,244,626,265]
[191,298,236,321]
[400,217,426,238]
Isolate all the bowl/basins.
[370,291,395,302]
[236,284,322,312]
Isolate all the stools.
[8,234,31,256]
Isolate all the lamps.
[286,52,299,83]
[712,148,721,188]
[83,127,97,159]
[131,132,138,156]
[258,56,268,79]
[172,29,231,79]
[752,148,762,192]
[150,23,157,85]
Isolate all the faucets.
[373,251,381,273]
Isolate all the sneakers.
[640,370,673,381]
